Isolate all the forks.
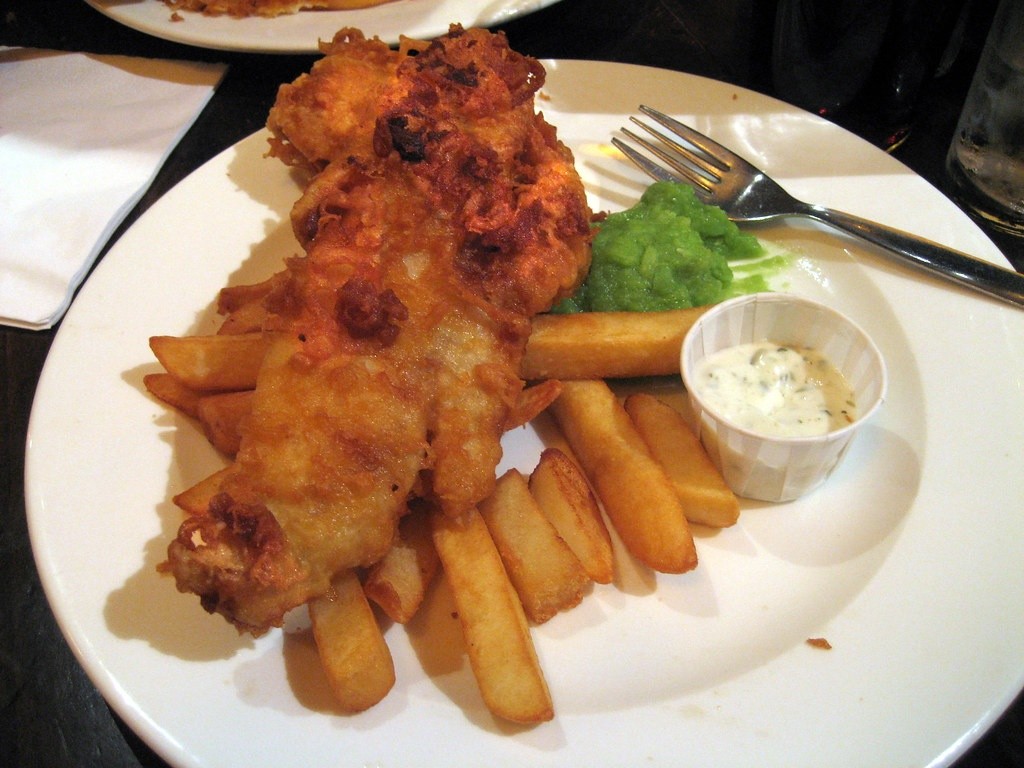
[612,105,1024,308]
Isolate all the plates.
[86,0,557,54]
[21,60,1022,768]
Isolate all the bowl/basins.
[680,292,888,503]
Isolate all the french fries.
[139,256,740,726]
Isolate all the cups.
[931,0,1024,238]
